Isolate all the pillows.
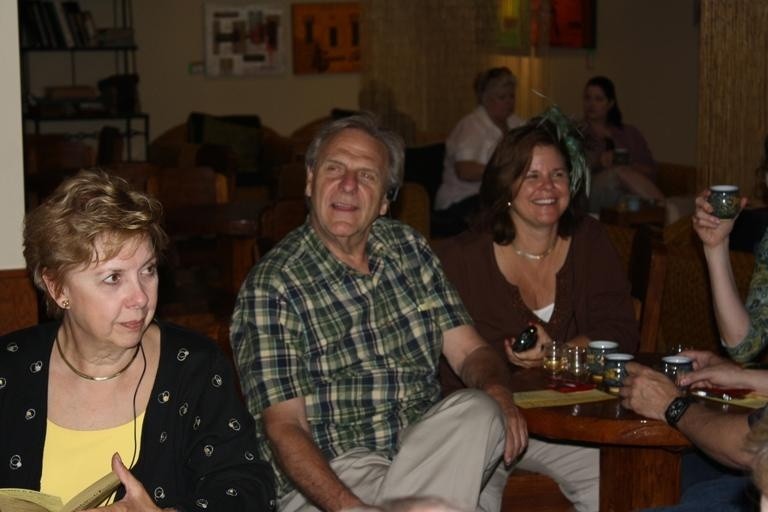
[188,112,272,188]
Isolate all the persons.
[429,124,640,512]
[691,186,768,363]
[571,74,664,211]
[429,67,525,238]
[620,350,767,512]
[229,114,528,512]
[0,169,278,512]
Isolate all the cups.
[566,346,586,375]
[662,356,693,384]
[604,353,634,393]
[541,342,565,374]
[586,340,619,379]
[709,185,740,218]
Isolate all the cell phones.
[513,325,538,353]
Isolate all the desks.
[158,203,275,297]
[511,342,764,512]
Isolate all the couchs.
[152,122,292,205]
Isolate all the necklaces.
[55,335,138,382]
[510,236,559,261]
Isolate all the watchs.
[664,396,697,428]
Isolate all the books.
[0,473,122,512]
[22,0,89,46]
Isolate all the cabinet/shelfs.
[19,1,149,164]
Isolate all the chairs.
[643,238,756,365]
[144,167,238,332]
